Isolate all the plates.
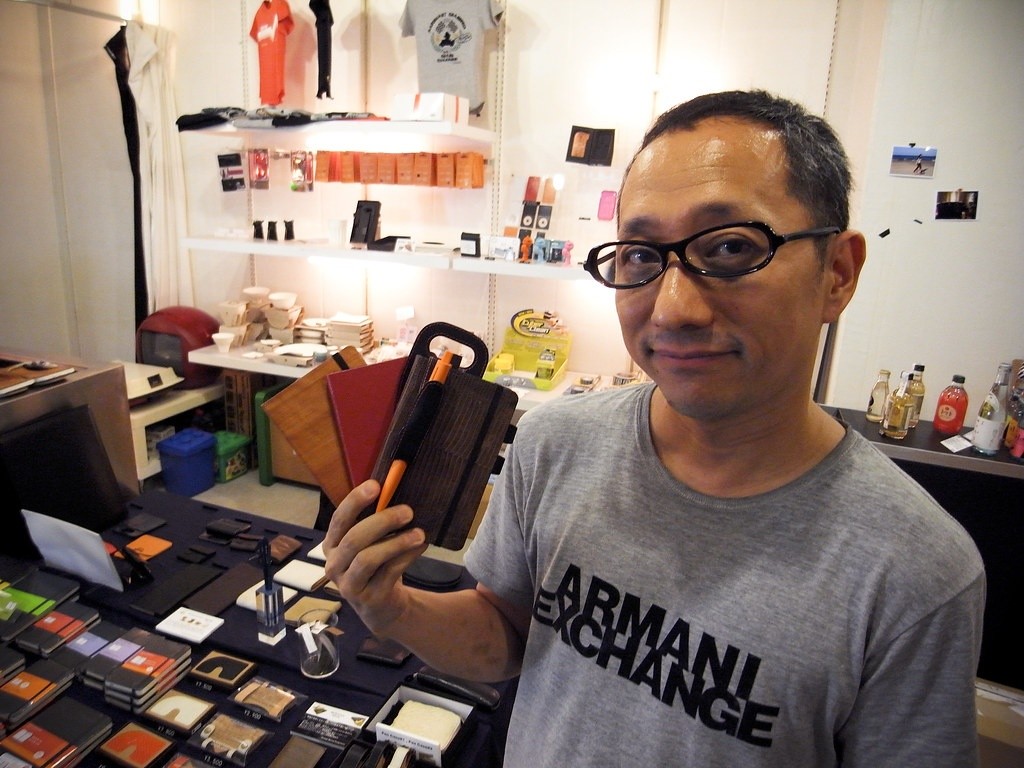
[272,341,327,356]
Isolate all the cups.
[212,332,234,353]
[312,349,327,367]
[219,323,247,347]
[296,607,343,679]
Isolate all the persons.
[323,91,987,768]
[913,153,925,173]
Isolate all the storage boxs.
[147,369,276,498]
[407,92,470,122]
[330,679,477,768]
[368,236,411,251]
[482,309,575,391]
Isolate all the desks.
[188,341,613,412]
[0,487,521,768]
[837,408,1024,691]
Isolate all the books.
[0,322,518,768]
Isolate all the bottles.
[538,348,554,379]
[865,365,926,439]
[493,352,515,376]
[972,362,1024,464]
[933,375,969,436]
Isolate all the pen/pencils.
[257,535,275,593]
[373,348,457,515]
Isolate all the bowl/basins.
[268,292,297,309]
[242,286,269,305]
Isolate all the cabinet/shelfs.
[129,381,225,494]
[179,121,593,280]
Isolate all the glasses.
[583,220,842,289]
